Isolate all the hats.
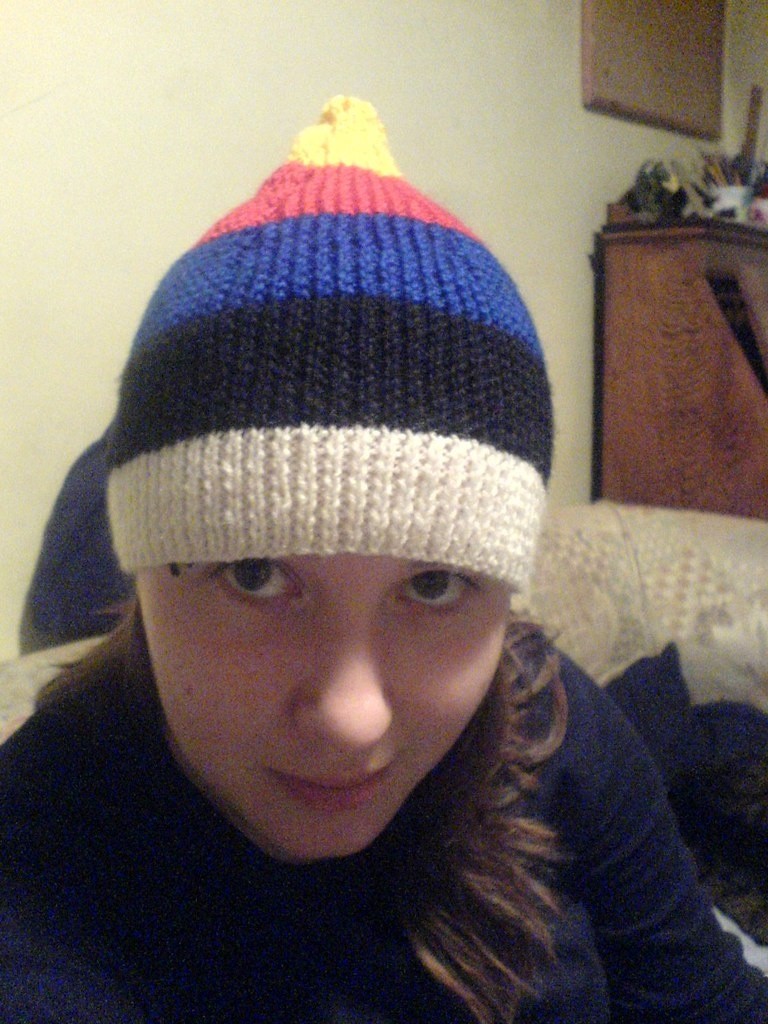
[104,94,555,590]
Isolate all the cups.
[713,186,755,223]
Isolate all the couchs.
[1,501,767,760]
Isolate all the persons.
[0,95,768,1023]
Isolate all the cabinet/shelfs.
[593,218,768,520]
